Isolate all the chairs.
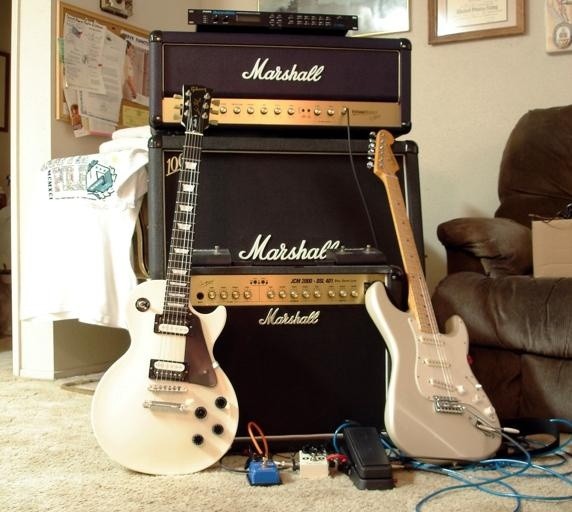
[431,103,571,438]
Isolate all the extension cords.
[300,448,330,478]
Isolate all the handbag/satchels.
[531,207,572,282]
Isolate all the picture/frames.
[426,0,527,48]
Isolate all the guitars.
[363,129,504,468]
[91,84,240,477]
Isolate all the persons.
[70,104,84,132]
[122,39,139,100]
